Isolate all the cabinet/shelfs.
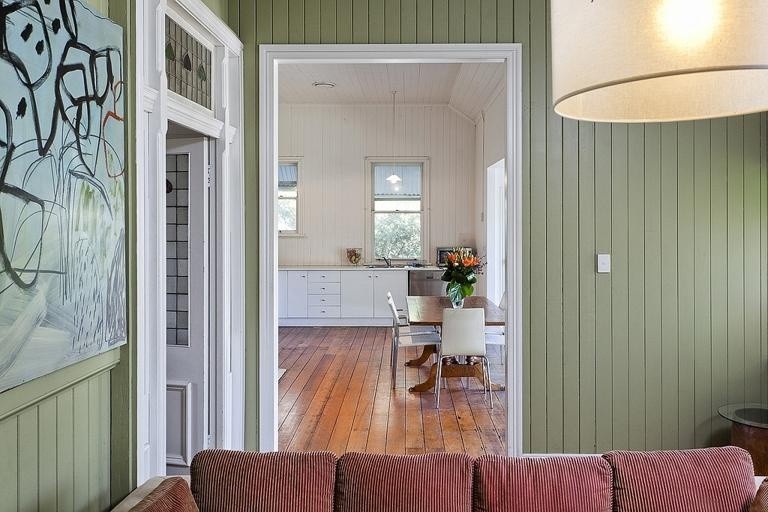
[278,271,340,319]
[341,270,408,319]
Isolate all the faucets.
[382,257,391,266]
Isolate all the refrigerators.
[409,270,449,296]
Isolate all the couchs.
[103,446,767,512]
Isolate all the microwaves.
[437,246,472,267]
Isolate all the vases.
[448,289,465,308]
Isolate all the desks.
[718,403,768,475]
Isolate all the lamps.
[544,1,768,126]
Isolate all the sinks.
[368,265,405,268]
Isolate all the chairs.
[386,290,507,410]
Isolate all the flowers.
[437,242,487,300]
